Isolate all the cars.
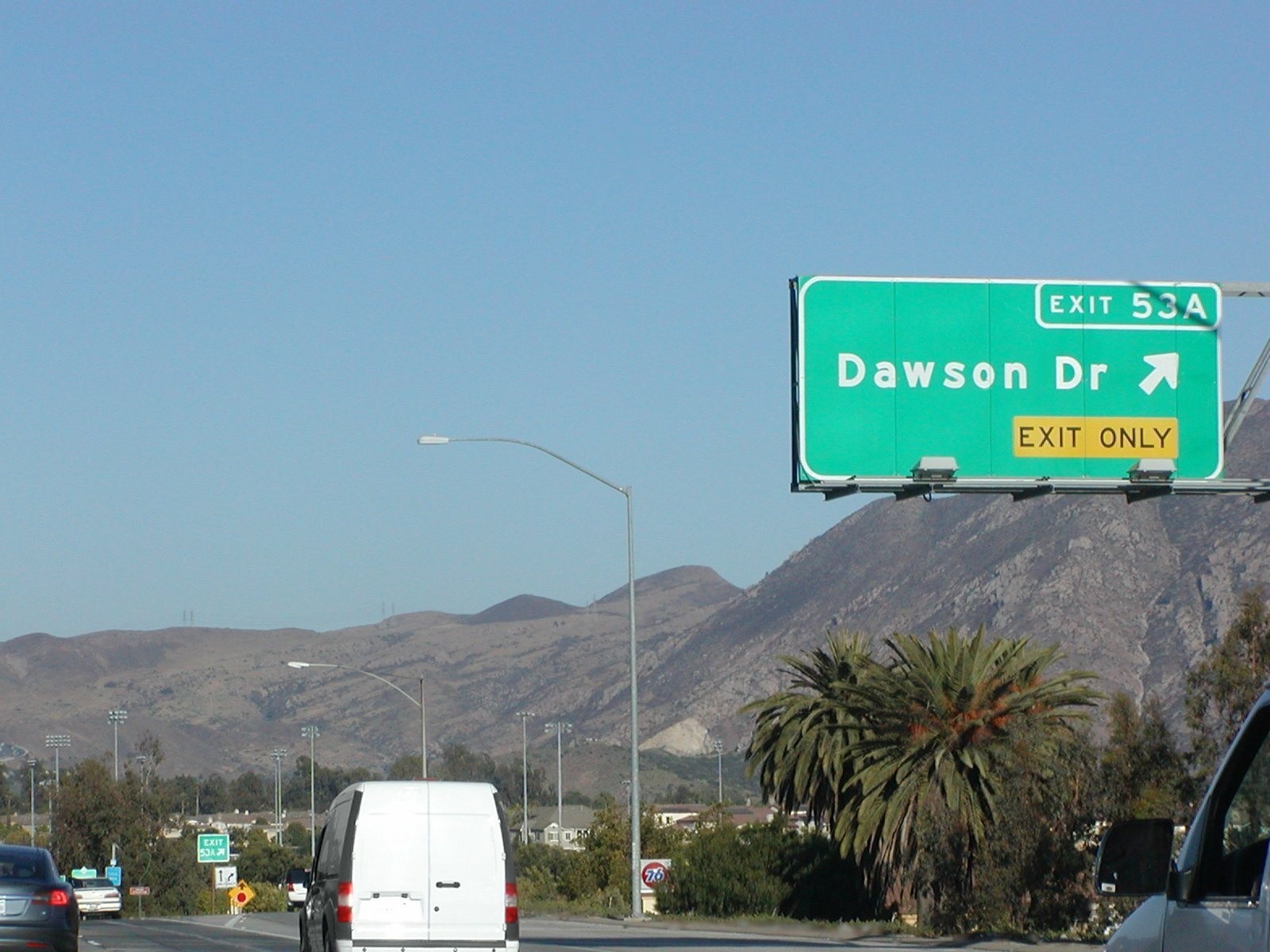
[70,879,123,919]
[1083,691,1270,952]
[0,844,84,952]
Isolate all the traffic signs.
[798,274,1227,483]
[197,834,231,865]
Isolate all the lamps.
[909,455,958,484]
[1128,457,1177,486]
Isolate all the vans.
[282,780,523,952]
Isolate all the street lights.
[288,660,429,780]
[135,755,147,817]
[713,738,724,827]
[545,719,571,850]
[108,711,129,782]
[27,754,38,847]
[300,724,321,859]
[45,734,73,803]
[413,434,647,921]
[270,748,286,848]
[516,711,536,845]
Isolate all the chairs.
[10,864,34,878]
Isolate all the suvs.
[279,867,314,912]
